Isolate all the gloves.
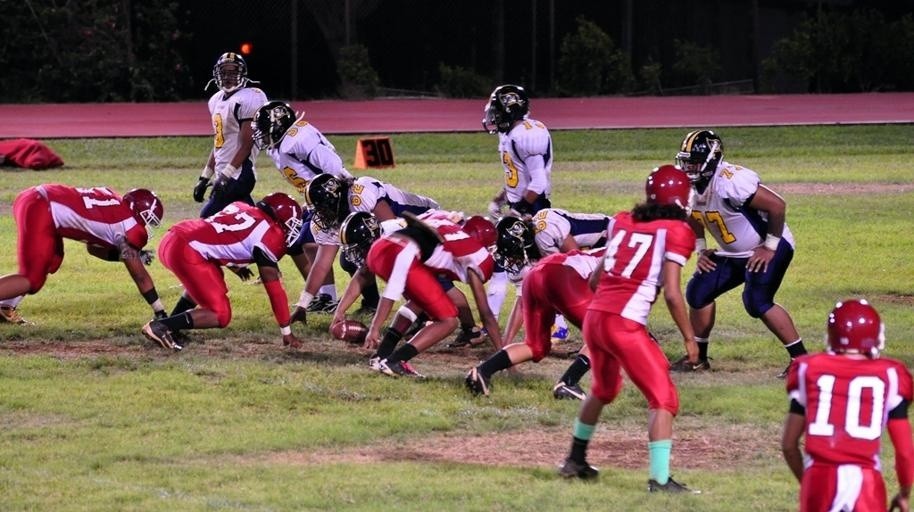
[505,208,521,218]
[205,172,229,200]
[488,202,501,220]
[137,249,155,265]
[192,177,209,202]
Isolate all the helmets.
[675,130,723,186]
[338,211,384,275]
[645,165,694,218]
[496,215,532,275]
[481,85,528,135]
[204,52,258,93]
[123,188,163,241]
[256,191,303,249]
[825,300,886,360]
[250,101,304,151]
[460,215,498,262]
[303,173,342,232]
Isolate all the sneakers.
[464,367,489,398]
[404,323,426,339]
[368,354,380,372]
[558,458,598,478]
[353,306,375,319]
[647,476,701,495]
[0,304,34,327]
[140,318,182,350]
[776,358,796,378]
[553,379,587,400]
[479,321,487,336]
[304,294,341,313]
[379,357,424,379]
[550,324,569,343]
[446,323,485,349]
[669,356,713,371]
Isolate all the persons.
[462,244,609,402]
[0,183,168,324]
[478,83,572,344]
[486,206,610,357]
[287,173,440,327]
[557,165,701,498]
[249,100,380,321]
[364,215,525,379]
[667,128,810,380]
[327,210,488,351]
[140,191,306,353]
[191,52,283,287]
[780,299,914,512]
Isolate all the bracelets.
[292,291,313,310]
[280,325,291,336]
[140,287,159,304]
[694,238,706,253]
[510,196,531,218]
[763,232,781,252]
[198,166,214,179]
[221,164,237,179]
[151,298,164,313]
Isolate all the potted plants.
[335,43,378,100]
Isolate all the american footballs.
[332,321,369,342]
